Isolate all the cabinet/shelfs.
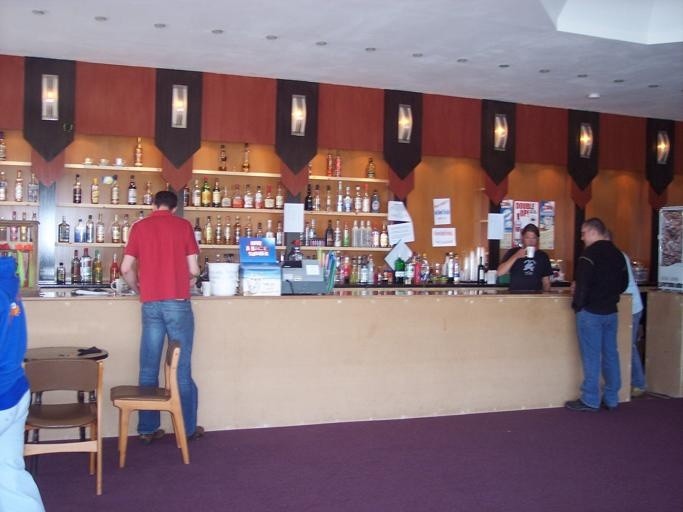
[0,220,40,288]
[53,163,395,252]
[0,160,40,205]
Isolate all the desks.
[23,347,109,479]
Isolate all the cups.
[114,158,122,165]
[487,270,496,285]
[524,245,535,260]
[84,157,92,164]
[99,159,109,165]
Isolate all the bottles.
[233,215,241,243]
[333,150,341,176]
[73,174,81,203]
[14,170,22,201]
[20,210,26,241]
[110,210,146,244]
[205,215,212,243]
[245,216,252,238]
[84,213,93,242]
[73,218,84,241]
[9,210,17,241]
[143,180,152,205]
[0,216,6,240]
[303,180,384,214]
[57,248,121,284]
[334,252,460,284]
[29,211,36,241]
[327,153,332,176]
[28,172,38,203]
[192,216,202,243]
[165,181,169,191]
[110,175,120,204]
[90,177,99,203]
[224,216,230,243]
[56,216,69,241]
[255,223,262,238]
[241,142,250,171]
[217,144,227,170]
[274,222,283,246]
[0,244,32,291]
[126,175,137,204]
[0,172,7,201]
[183,186,189,207]
[264,219,274,238]
[192,176,284,209]
[96,212,103,241]
[134,137,144,166]
[0,131,5,161]
[366,157,376,178]
[197,252,236,288]
[304,217,389,248]
[476,255,485,283]
[215,215,221,242]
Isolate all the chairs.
[110,340,189,469]
[21,360,104,495]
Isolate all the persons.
[0,255,46,512]
[118,191,206,445]
[564,218,627,411]
[495,222,553,293]
[603,225,646,401]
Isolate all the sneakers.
[565,398,616,412]
[187,426,204,441]
[138,429,165,446]
[631,385,645,397]
